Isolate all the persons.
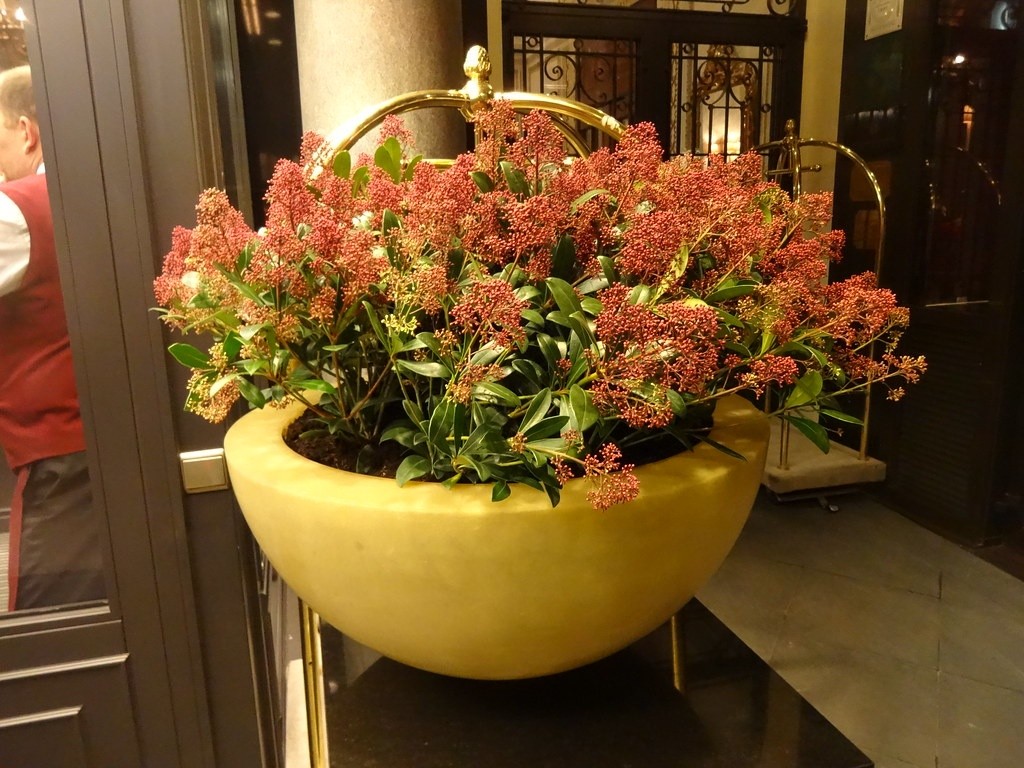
[0,62,107,612]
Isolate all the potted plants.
[149,99,929,681]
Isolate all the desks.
[298,590,877,768]
